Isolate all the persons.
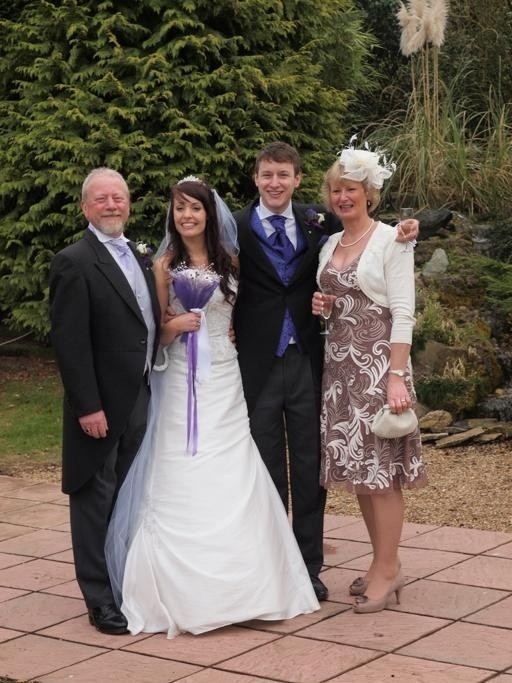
[312,142,429,615]
[104,174,322,642]
[48,167,239,637]
[162,142,420,605]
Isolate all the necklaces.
[337,217,375,247]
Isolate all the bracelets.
[387,368,405,378]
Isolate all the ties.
[266,215,296,264]
[111,239,135,272]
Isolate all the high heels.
[353,567,405,614]
[349,576,370,596]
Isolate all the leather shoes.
[310,574,329,601]
[88,603,129,634]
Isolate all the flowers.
[166,259,225,345]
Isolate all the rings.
[401,400,406,402]
[195,321,201,326]
[85,428,91,434]
